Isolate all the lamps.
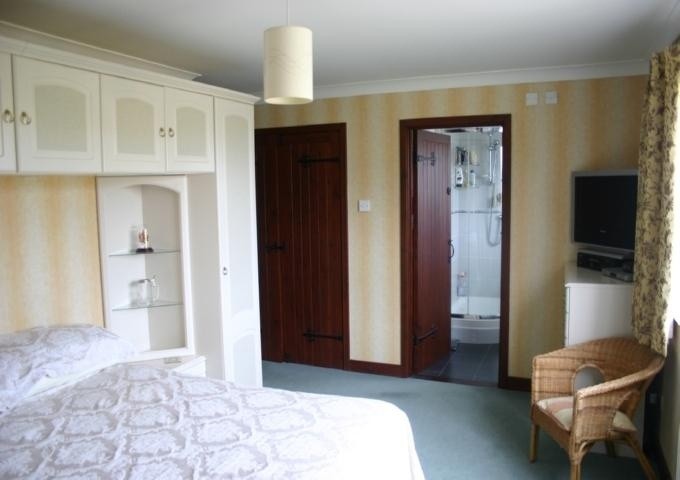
[259,0,315,105]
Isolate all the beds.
[0,327,427,480]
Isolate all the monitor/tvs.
[570,170,638,260]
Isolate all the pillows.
[0,324,131,412]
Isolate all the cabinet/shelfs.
[100,70,215,175]
[563,263,646,458]
[188,93,264,389]
[0,34,103,177]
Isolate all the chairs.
[529,335,666,479]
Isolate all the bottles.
[137,224,153,252]
